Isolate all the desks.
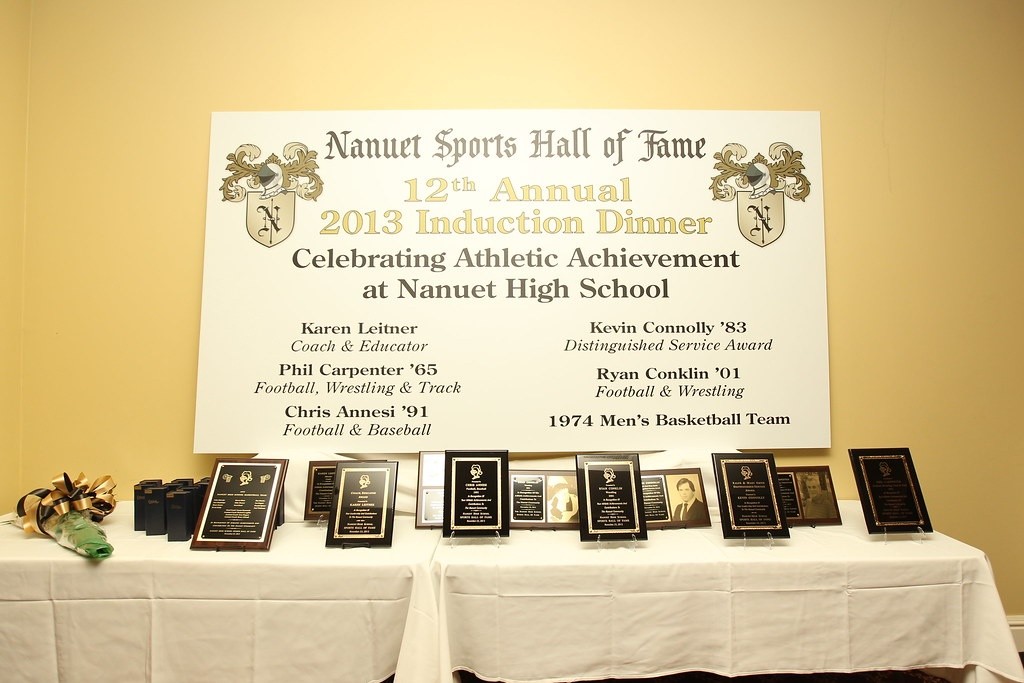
[0,499,1024,683]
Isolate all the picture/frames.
[845,445,932,532]
[444,448,509,537]
[190,457,290,551]
[575,454,649,544]
[303,460,389,520]
[640,467,711,528]
[712,452,791,540]
[325,461,400,548]
[416,449,446,529]
[509,472,578,529]
[778,465,842,527]
